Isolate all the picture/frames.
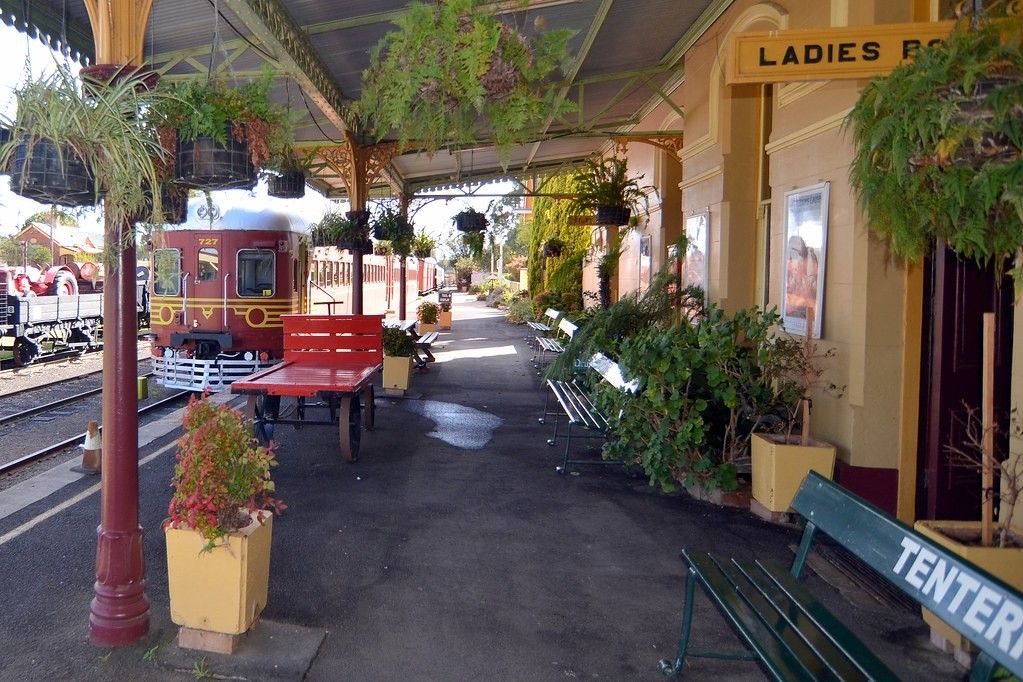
[683,210,711,325]
[638,236,653,303]
[778,181,831,338]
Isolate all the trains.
[143,202,443,397]
[0,261,151,368]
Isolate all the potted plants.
[380,324,414,390]
[437,302,454,328]
[742,306,845,513]
[415,300,438,335]
[164,387,286,636]
[1,0,657,258]
[913,397,1023,650]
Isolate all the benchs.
[656,468,1023,682]
[530,318,580,378]
[524,308,560,350]
[539,348,642,476]
[399,319,439,368]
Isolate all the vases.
[461,287,467,291]
